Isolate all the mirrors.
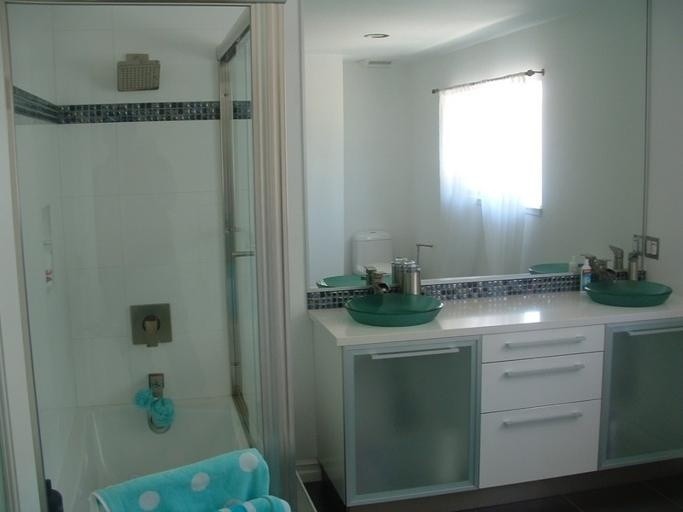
[340,28,649,295]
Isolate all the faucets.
[608,244,623,270]
[578,252,595,271]
[360,265,378,285]
[628,249,639,280]
[368,271,391,295]
[595,257,612,281]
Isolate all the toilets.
[351,230,393,275]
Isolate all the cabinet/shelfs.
[478,324,605,491]
[314,320,481,509]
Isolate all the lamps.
[599,320,682,472]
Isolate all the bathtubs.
[56,396,250,512]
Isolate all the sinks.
[343,292,444,325]
[528,262,595,272]
[133,380,174,434]
[316,274,393,289]
[583,279,672,306]
